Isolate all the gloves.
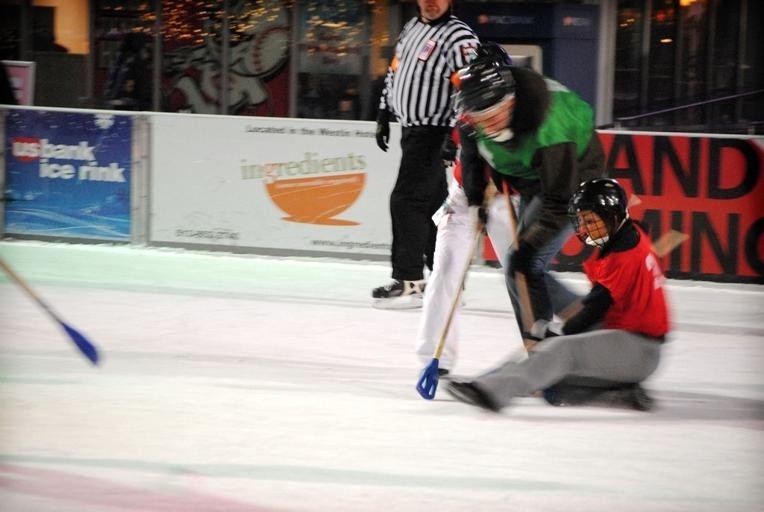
[543,319,563,338]
[375,113,389,152]
[440,126,457,161]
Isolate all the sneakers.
[372,278,424,297]
[441,379,501,411]
[627,380,652,411]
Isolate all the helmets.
[567,178,627,250]
[449,53,517,144]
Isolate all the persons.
[367,1,487,298]
[415,42,522,376]
[457,64,606,358]
[445,177,673,414]
